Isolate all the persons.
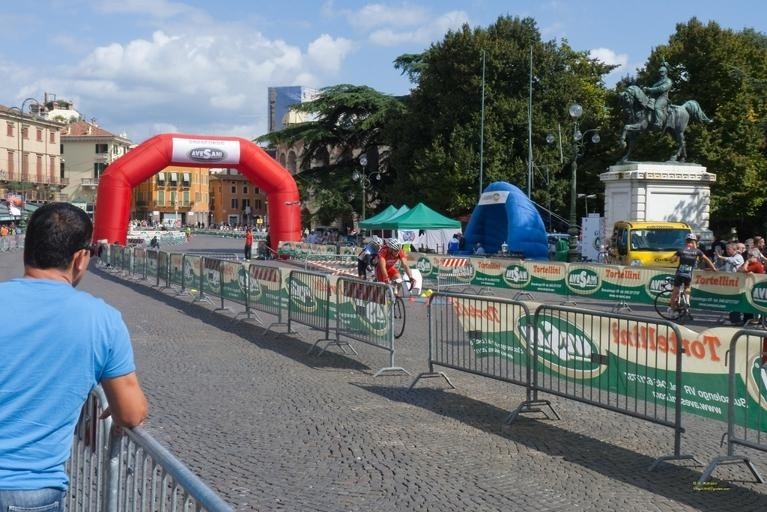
[356,237,383,280]
[0,202,150,512]
[447,234,459,251]
[705,235,766,327]
[0,220,23,254]
[126,219,267,242]
[472,242,485,254]
[242,229,253,262]
[374,237,416,305]
[300,228,348,243]
[546,241,556,260]
[456,232,465,251]
[662,233,718,319]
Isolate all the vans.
[545,232,583,260]
[606,219,701,269]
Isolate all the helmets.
[685,233,697,240]
[370,237,383,247]
[386,238,401,250]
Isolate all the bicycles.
[388,278,414,339]
[653,273,693,326]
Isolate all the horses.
[616,85,713,161]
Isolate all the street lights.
[352,155,382,223]
[546,103,601,264]
[5,96,67,233]
